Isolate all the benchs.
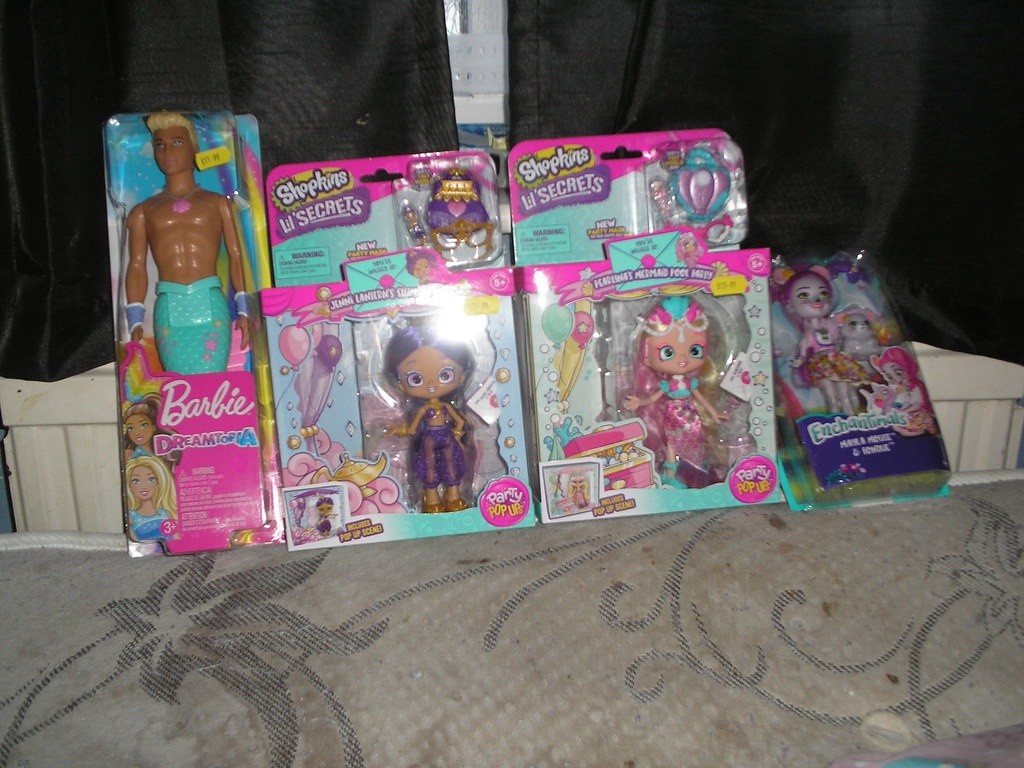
[0,356,1024,768]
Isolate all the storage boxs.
[104,113,954,560]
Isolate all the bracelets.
[234,291,249,319]
[125,302,145,334]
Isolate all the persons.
[621,295,731,490]
[125,110,249,375]
[774,265,873,416]
[383,325,475,514]
